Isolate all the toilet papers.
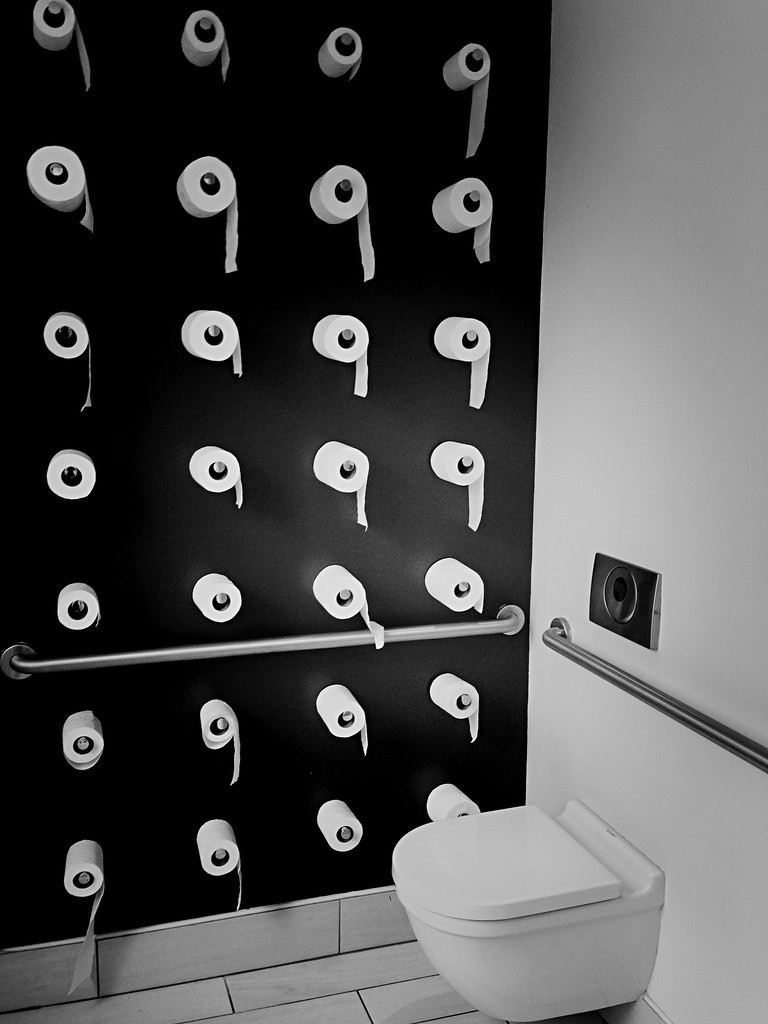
[311,564,386,650]
[175,155,241,274]
[315,800,365,853]
[311,314,370,399]
[424,557,485,615]
[431,175,495,266]
[196,819,243,913]
[198,699,242,786]
[26,143,96,234]
[191,572,243,623]
[63,839,106,997]
[182,8,232,83]
[430,672,481,744]
[45,448,97,501]
[188,445,244,510]
[61,708,105,772]
[312,440,370,533]
[430,440,486,533]
[317,25,365,83]
[443,41,493,160]
[314,684,369,757]
[31,1,93,92]
[181,309,244,379]
[56,581,101,631]
[426,783,482,823]
[308,163,378,284]
[43,312,92,413]
[434,314,493,410]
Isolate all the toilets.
[389,797,665,1022]
